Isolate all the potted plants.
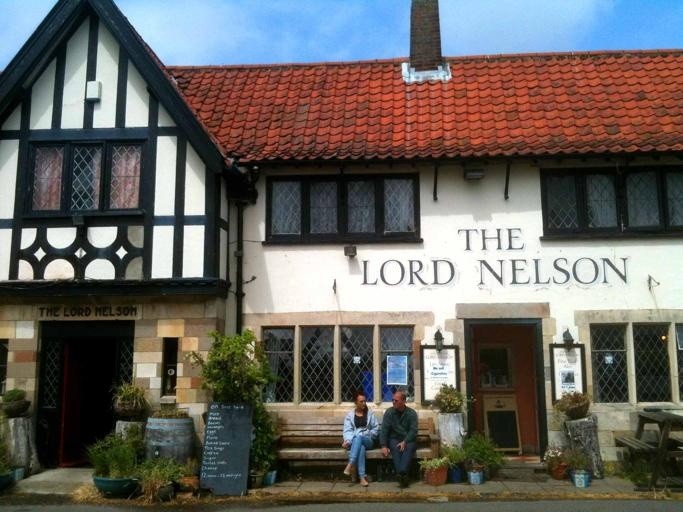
[0,388,32,417]
[0,430,18,490]
[419,431,504,485]
[568,446,593,488]
[86,382,200,502]
[553,391,591,420]
[264,454,278,485]
[434,383,466,413]
[546,441,571,480]
[248,453,264,488]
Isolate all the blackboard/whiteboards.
[483,394,523,455]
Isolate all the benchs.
[274,415,441,482]
[614,434,659,452]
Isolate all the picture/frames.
[420,345,460,406]
[549,343,587,406]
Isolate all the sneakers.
[343,469,351,477]
[359,480,369,487]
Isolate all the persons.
[379,390,418,488]
[342,393,380,487]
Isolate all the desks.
[630,411,683,491]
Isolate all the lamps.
[73,214,87,236]
[434,329,444,352]
[563,328,574,352]
[85,80,101,103]
[463,161,485,180]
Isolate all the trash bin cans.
[200,401,253,495]
